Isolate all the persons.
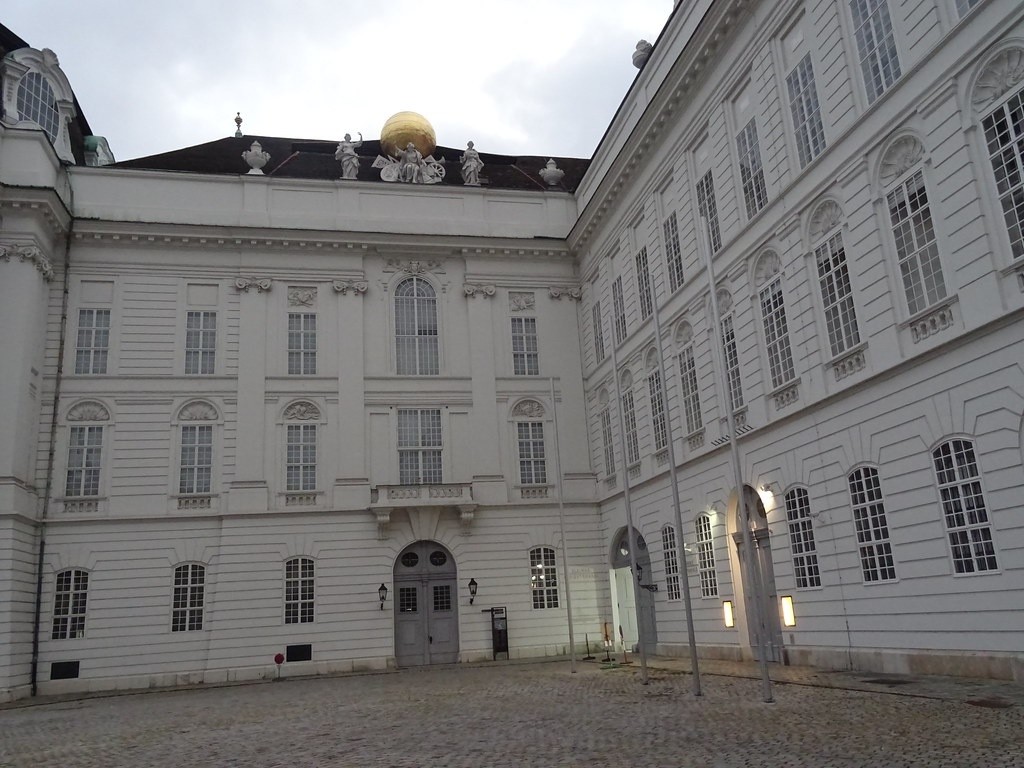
[395,142,423,183]
[459,141,484,185]
[335,132,363,179]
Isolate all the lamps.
[780,595,796,627]
[630,563,657,592]
[378,583,387,610]
[723,601,735,629]
[759,481,781,492]
[468,577,478,605]
